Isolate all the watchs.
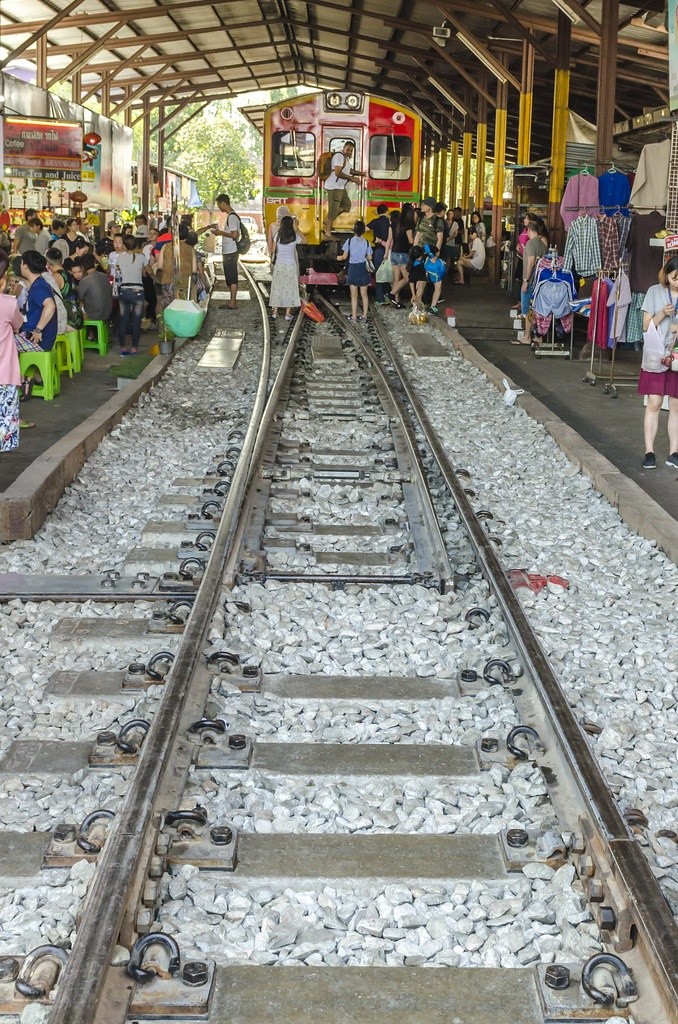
[524,278,529,281]
[35,328,43,333]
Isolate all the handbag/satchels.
[365,245,448,284]
[272,234,280,265]
[300,297,325,322]
[641,319,671,373]
[62,294,83,331]
[112,280,120,296]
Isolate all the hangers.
[546,272,565,283]
[580,204,623,221]
[608,162,617,173]
[599,263,624,281]
[580,162,588,175]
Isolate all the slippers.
[509,339,530,345]
[219,304,238,309]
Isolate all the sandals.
[18,376,34,402]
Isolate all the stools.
[20,351,59,398]
[80,320,109,355]
[51,330,84,379]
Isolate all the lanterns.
[70,192,88,204]
[84,132,101,146]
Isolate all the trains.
[261,89,425,294]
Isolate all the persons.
[268,196,549,345]
[0,209,216,454]
[637,257,678,469]
[210,193,242,309]
[322,142,367,241]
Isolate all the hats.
[469,227,476,232]
[419,197,436,209]
[133,225,149,238]
[107,221,120,227]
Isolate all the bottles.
[102,255,108,270]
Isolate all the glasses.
[468,232,475,235]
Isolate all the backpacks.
[227,212,251,255]
[317,152,345,182]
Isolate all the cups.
[669,311,678,333]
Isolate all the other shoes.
[130,348,136,356]
[285,315,293,320]
[428,307,438,316]
[323,234,340,241]
[453,279,464,284]
[323,220,336,231]
[120,346,126,357]
[437,295,444,303]
[358,316,366,321]
[396,304,406,308]
[642,453,656,468]
[383,292,400,308]
[666,452,678,468]
[272,313,278,318]
[347,316,356,321]
